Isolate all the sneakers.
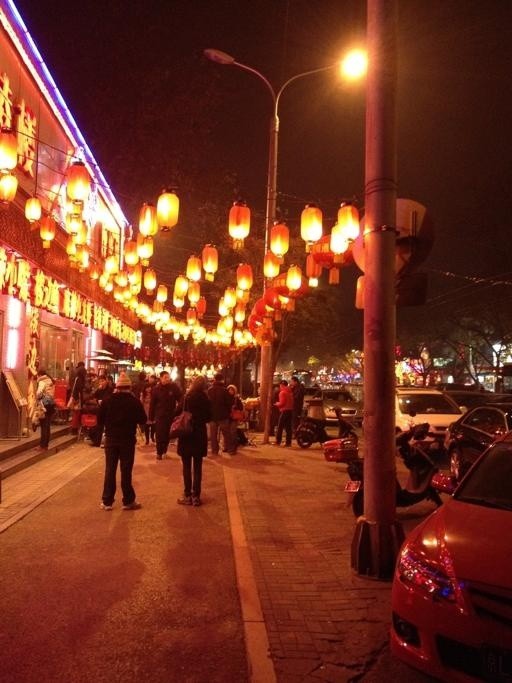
[190,494,200,506]
[122,498,141,510]
[97,501,112,511]
[176,495,192,506]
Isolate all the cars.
[395,385,462,433]
[306,387,363,430]
[443,401,511,483]
[445,388,512,410]
[301,385,322,411]
[385,428,512,682]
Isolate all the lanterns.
[0,125,359,349]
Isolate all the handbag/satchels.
[168,400,193,439]
[27,396,47,427]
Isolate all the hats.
[116,372,130,388]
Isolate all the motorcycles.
[322,421,444,520]
[293,404,359,449]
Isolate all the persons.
[36,361,306,512]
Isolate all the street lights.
[199,40,371,433]
